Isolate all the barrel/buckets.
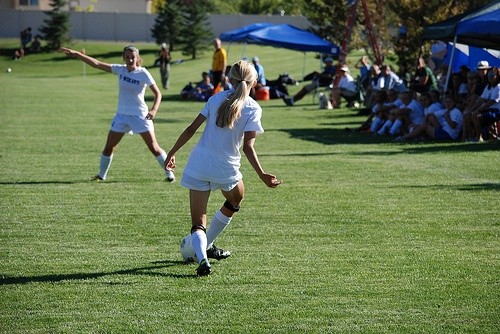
[255,86,270,100]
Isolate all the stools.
[256,87,269,100]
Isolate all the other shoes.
[90,176,103,182]
[166,177,175,182]
[282,94,293,105]
[207,243,231,261]
[195,264,211,277]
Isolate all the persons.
[60,47,176,183]
[181,37,500,146]
[13,27,41,59]
[164,60,283,277]
[156,43,170,91]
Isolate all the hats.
[324,57,333,62]
[340,67,348,72]
[476,61,491,69]
[379,65,390,71]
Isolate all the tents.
[218,22,340,84]
[416,0,500,92]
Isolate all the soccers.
[180,234,199,262]
